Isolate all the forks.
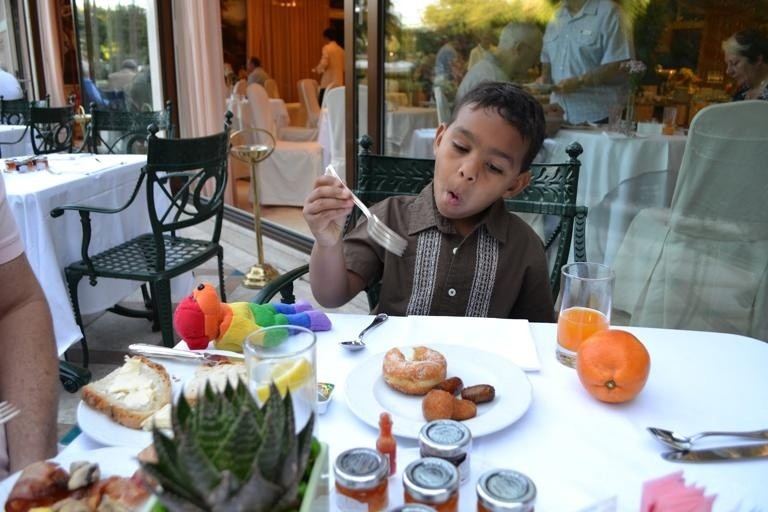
[324,165,408,256]
[76,363,311,460]
[0,399,22,430]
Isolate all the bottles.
[335,407,536,512]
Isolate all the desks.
[385,105,436,157]
[0,152,170,357]
[225,99,290,180]
[4,124,40,157]
[553,122,688,310]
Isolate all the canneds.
[333,418,535,512]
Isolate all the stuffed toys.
[172,283,333,352]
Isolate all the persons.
[0,65,25,126]
[81,58,151,115]
[534,2,632,127]
[0,175,59,474]
[303,83,558,324]
[721,30,768,101]
[247,57,268,90]
[311,28,344,107]
[454,21,543,109]
[434,31,466,100]
[467,28,499,75]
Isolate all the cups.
[606,103,622,130]
[661,107,678,134]
[555,261,617,371]
[242,324,317,447]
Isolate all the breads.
[78,354,246,431]
[423,377,495,422]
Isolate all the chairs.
[0,79,768,392]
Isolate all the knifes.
[655,444,768,465]
[125,339,243,362]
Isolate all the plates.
[0,450,162,512]
[344,346,532,441]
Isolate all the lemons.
[256,358,312,403]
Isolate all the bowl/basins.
[315,381,337,416]
[544,117,564,138]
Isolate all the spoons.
[339,311,388,353]
[642,420,768,449]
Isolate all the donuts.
[384,345,445,394]
